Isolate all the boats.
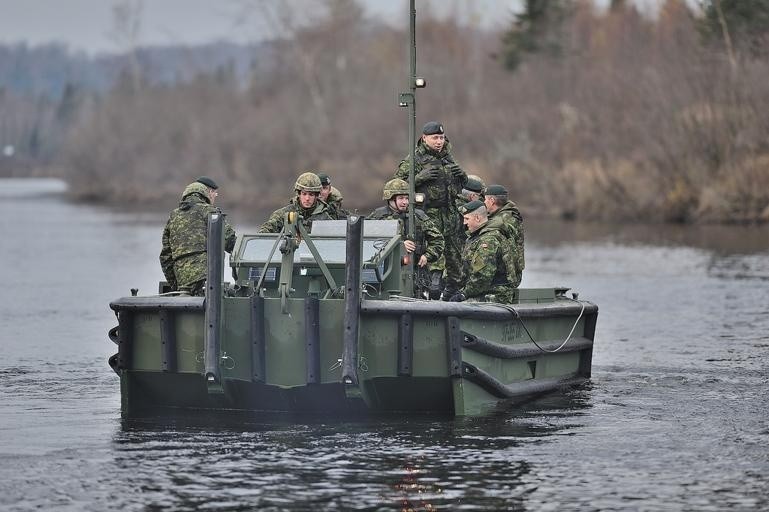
[106,1,597,417]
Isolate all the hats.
[197,176,218,190]
[457,174,508,217]
[318,173,331,187]
[421,122,445,136]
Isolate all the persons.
[197,176,218,203]
[159,182,237,297]
[393,121,468,301]
[366,174,526,304]
[257,172,352,237]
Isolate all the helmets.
[180,182,212,204]
[293,172,323,194]
[382,179,411,201]
